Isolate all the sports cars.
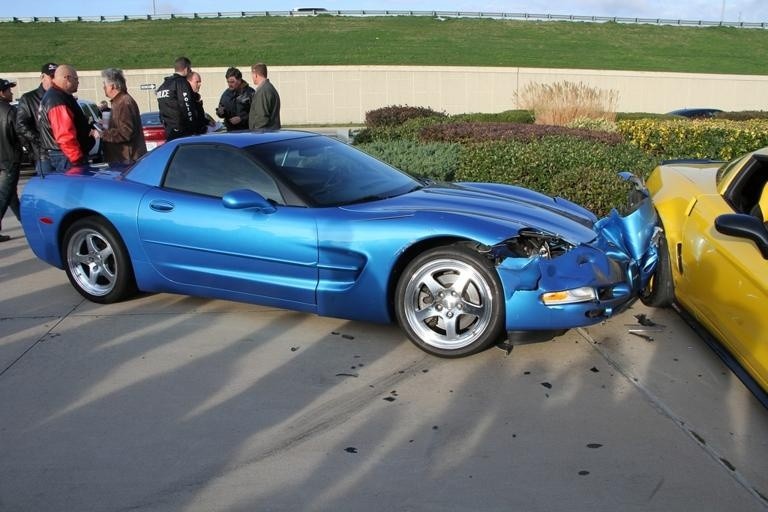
[17,131,659,356]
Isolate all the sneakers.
[0,235,10,242]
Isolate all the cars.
[665,108,725,120]
[79,102,102,156]
[142,112,224,152]
[640,146,768,412]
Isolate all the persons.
[100,100,112,113]
[187,71,217,137]
[248,62,281,131]
[155,57,202,143]
[90,66,148,163]
[15,62,59,162]
[0,78,24,242]
[215,66,256,132]
[37,63,97,175]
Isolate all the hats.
[42,62,59,77]
[0,79,17,89]
